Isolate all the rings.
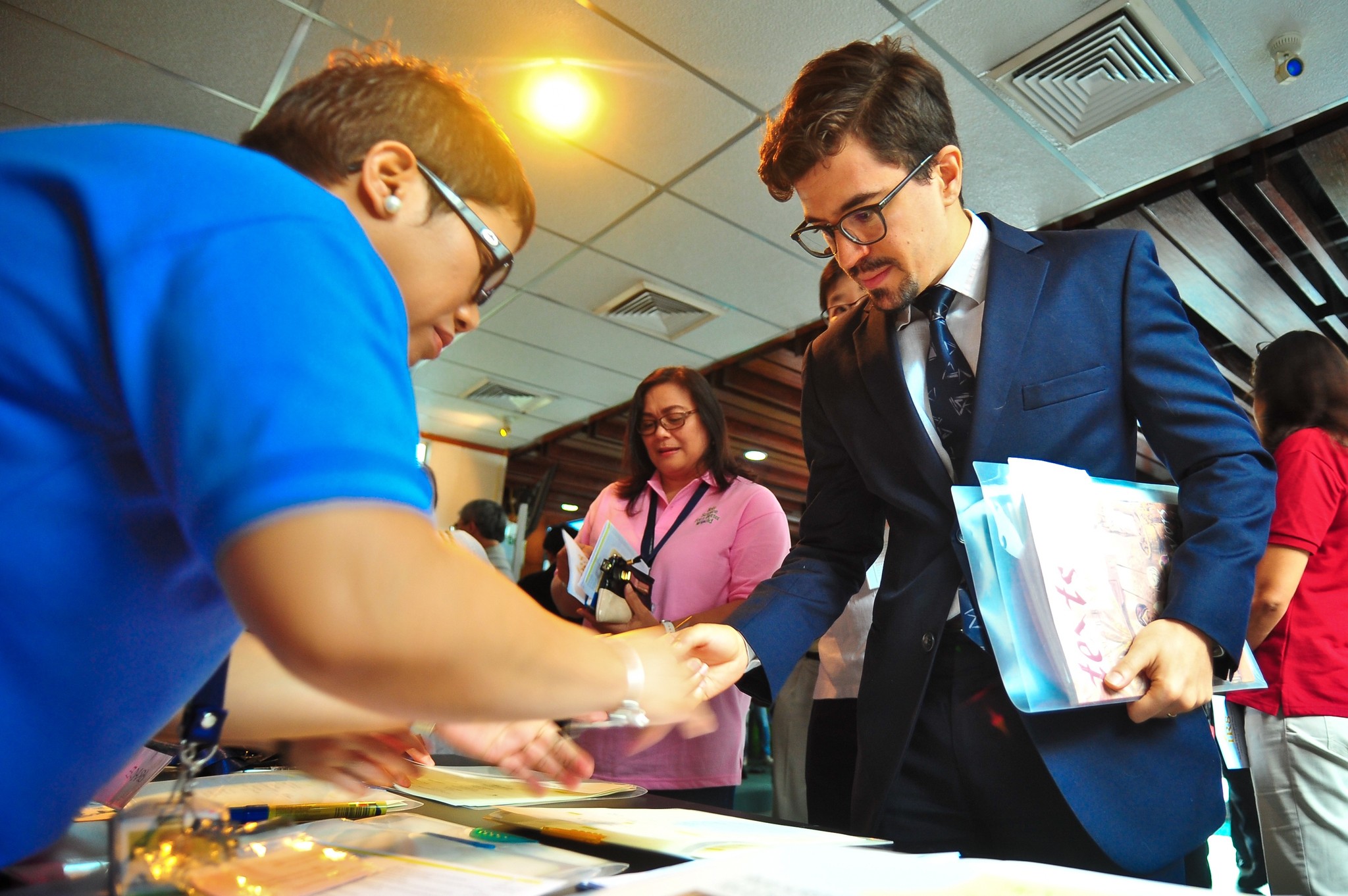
[1167,713,1177,719]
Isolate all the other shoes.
[1238,864,1267,890]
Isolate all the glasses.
[635,408,699,436]
[820,293,869,327]
[789,154,935,258]
[342,158,515,306]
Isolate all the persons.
[406,463,492,755]
[454,499,517,585]
[0,39,710,896]
[512,524,590,625]
[551,367,791,809]
[807,253,886,837]
[1238,330,1348,896]
[667,37,1278,889]
[1199,692,1267,896]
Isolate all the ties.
[910,284,992,655]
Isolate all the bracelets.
[606,635,643,727]
[658,619,675,635]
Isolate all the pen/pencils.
[228,802,386,823]
